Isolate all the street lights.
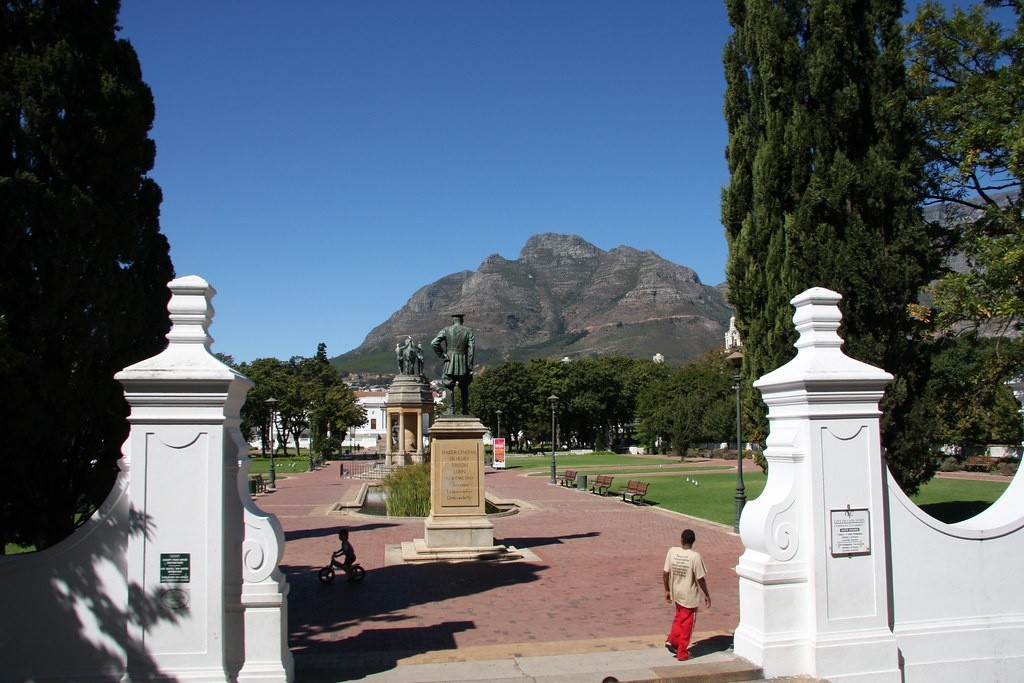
[265,396,277,488]
[724,347,747,534]
[548,394,559,484]
[495,409,503,438]
[306,411,314,472]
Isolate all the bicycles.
[318,553,366,583]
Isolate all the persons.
[394,337,425,376]
[662,528,711,661]
[431,313,476,416]
[392,421,399,437]
[331,528,356,582]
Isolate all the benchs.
[556,470,578,487]
[251,475,272,493]
[617,480,650,506]
[591,475,614,496]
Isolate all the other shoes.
[666,641,677,654]
[346,577,353,582]
[679,651,691,661]
